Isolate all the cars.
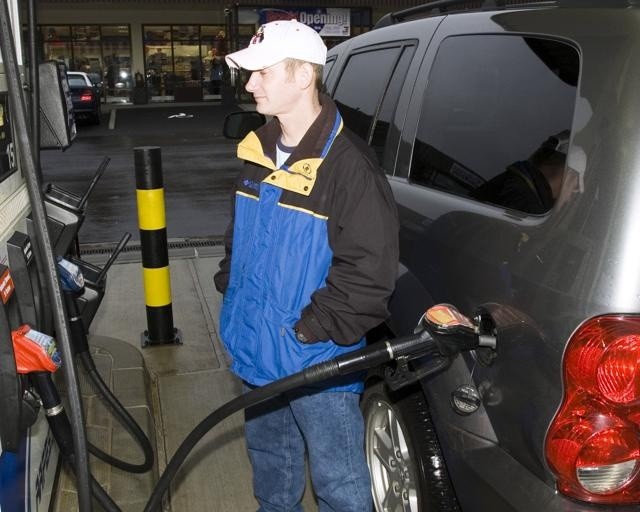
[65,70,105,126]
[112,65,154,92]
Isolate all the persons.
[213,19,402,511]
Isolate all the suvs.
[221,2,638,511]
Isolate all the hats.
[225,18,326,71]
